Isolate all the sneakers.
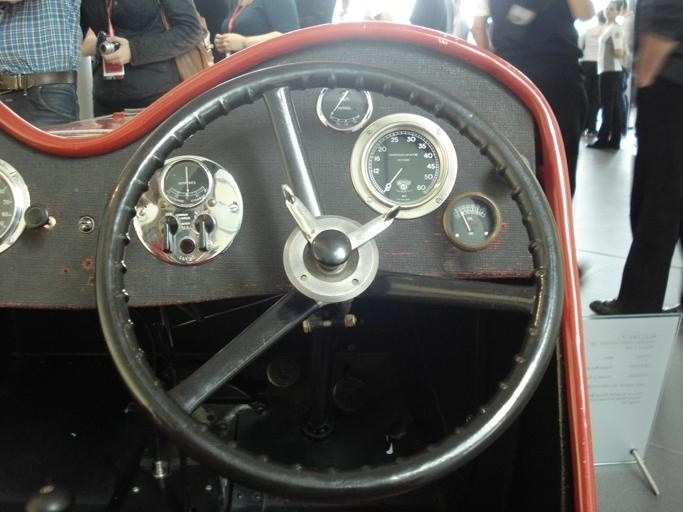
[589,300,616,314]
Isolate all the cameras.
[96,30,121,55]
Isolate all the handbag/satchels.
[172,18,215,79]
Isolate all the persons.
[0,0,83,131]
[468,1,596,279]
[295,0,337,29]
[213,0,300,57]
[589,2,682,316]
[410,0,460,35]
[193,0,238,63]
[580,0,635,150]
[79,0,203,117]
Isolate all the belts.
[0,70,75,90]
